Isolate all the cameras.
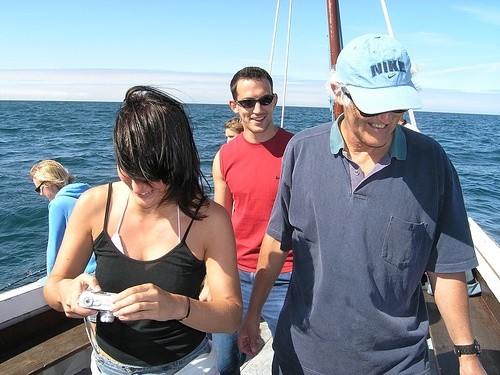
[78,290,121,322]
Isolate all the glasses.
[342,88,409,118]
[35,182,44,193]
[235,94,274,108]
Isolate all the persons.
[212,67,294,375]
[238,32,488,375]
[29,159,102,345]
[224,117,244,144]
[43,85,243,375]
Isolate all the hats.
[336,35,422,114]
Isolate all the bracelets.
[454,339,482,357]
[176,296,191,323]
